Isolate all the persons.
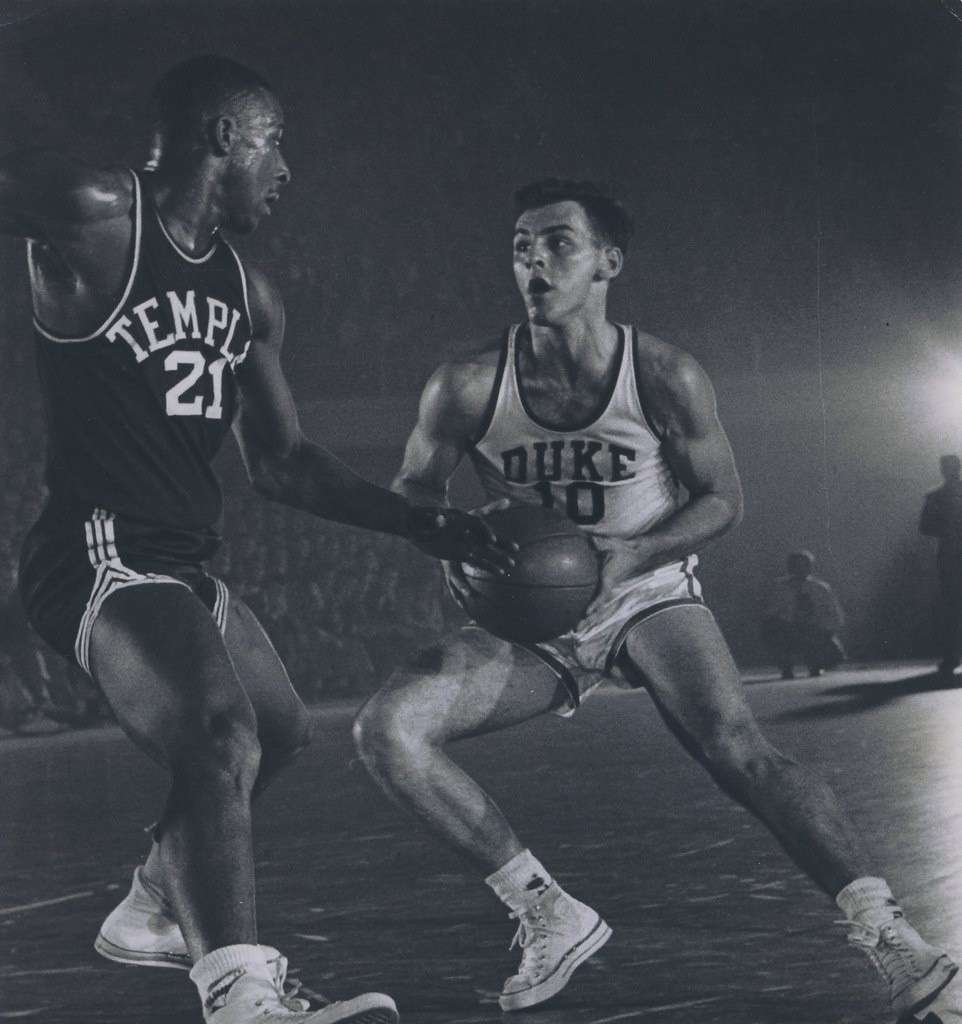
[917,455,962,677]
[1,236,743,737]
[353,180,962,1023]
[759,550,847,678]
[0,55,519,1024]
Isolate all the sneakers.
[832,916,958,1019]
[498,879,614,1012]
[205,956,400,1024]
[94,864,194,971]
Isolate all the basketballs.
[453,502,602,646]
